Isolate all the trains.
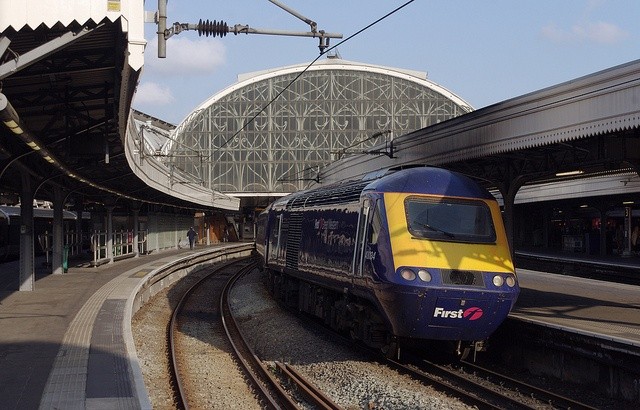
[254,164,521,362]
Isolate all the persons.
[186,226,200,250]
